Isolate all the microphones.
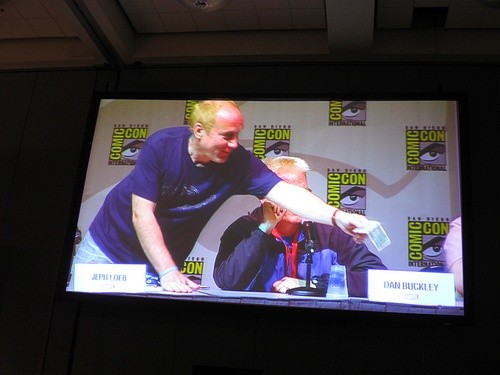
[301,218,317,253]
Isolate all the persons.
[66,101,369,294]
[444,216,464,297]
[213,157,389,297]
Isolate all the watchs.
[311,278,320,288]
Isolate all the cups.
[326,264,349,299]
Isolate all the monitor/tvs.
[192,365,263,375]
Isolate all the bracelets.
[332,209,341,227]
[158,266,178,278]
